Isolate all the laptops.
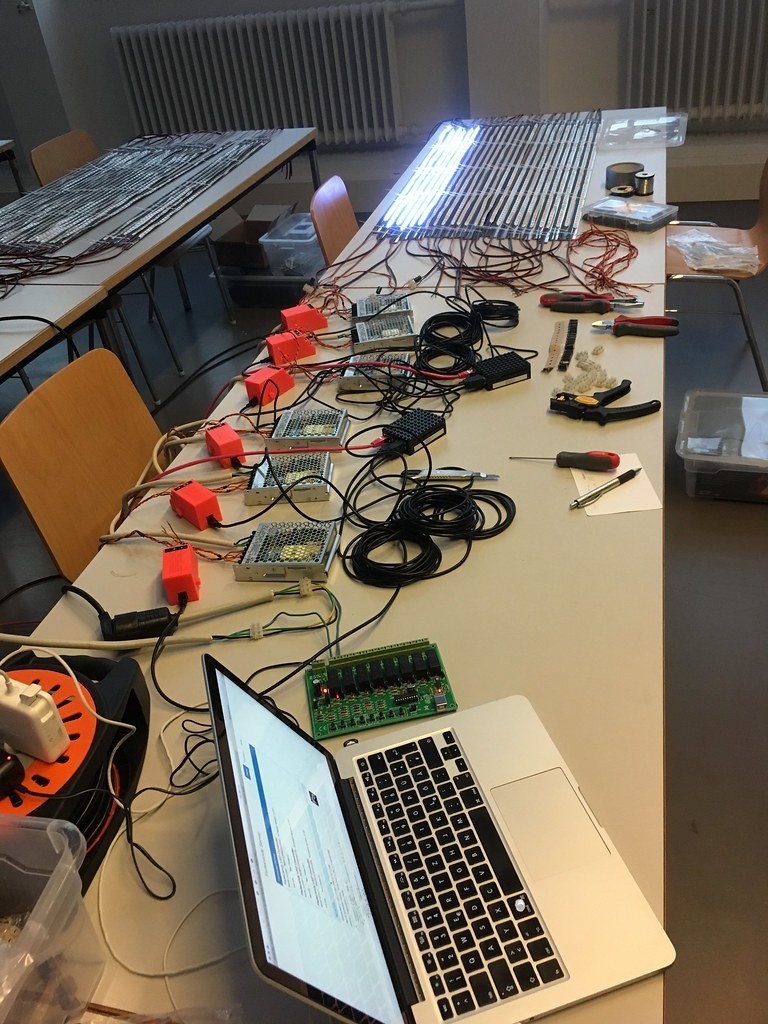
[202,652,677,1024]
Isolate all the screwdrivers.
[507,451,621,471]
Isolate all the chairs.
[667,157,768,392]
[28,127,99,188]
[309,175,360,269]
[0,347,171,582]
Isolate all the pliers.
[591,314,680,337]
[538,291,645,312]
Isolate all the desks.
[0,105,668,1024]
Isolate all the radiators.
[110,0,402,149]
[620,0,768,138]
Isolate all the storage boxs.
[209,265,326,306]
[208,205,296,268]
[0,815,106,1024]
[674,389,768,504]
[259,213,325,277]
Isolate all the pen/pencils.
[568,467,644,510]
